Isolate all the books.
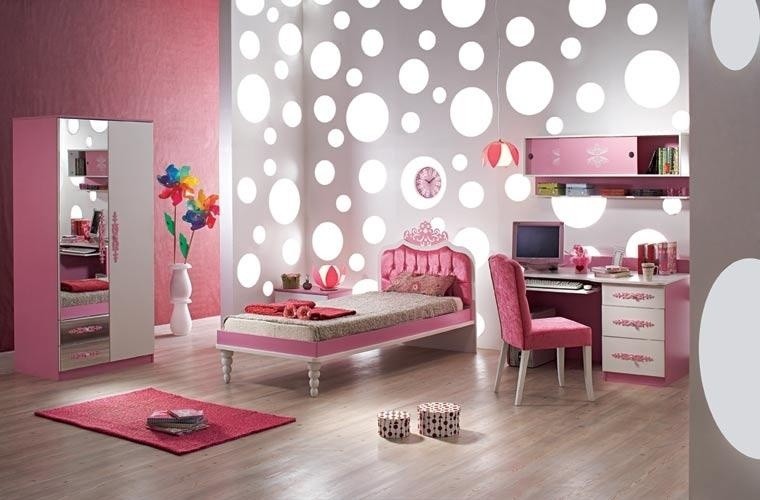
[645,145,679,175]
[75,157,85,176]
[591,265,631,279]
[144,409,210,436]
[60,234,84,243]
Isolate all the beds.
[60,277,110,344]
[217,221,477,397]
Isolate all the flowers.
[157,165,219,262]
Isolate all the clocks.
[415,167,441,199]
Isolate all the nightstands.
[273,287,352,303]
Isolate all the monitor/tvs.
[511,220,565,272]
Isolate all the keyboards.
[525,279,583,290]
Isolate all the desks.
[60,241,108,282]
[507,274,691,386]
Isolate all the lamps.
[480,0,519,169]
[312,265,346,291]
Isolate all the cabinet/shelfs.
[68,150,108,191]
[12,115,155,382]
[523,133,689,199]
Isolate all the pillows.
[383,272,456,299]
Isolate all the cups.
[640,262,659,283]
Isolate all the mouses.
[584,285,592,291]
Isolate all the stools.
[418,403,460,437]
[377,410,410,439]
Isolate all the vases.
[169,262,193,336]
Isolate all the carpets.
[35,387,296,455]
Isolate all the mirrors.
[57,118,111,372]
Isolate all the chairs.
[489,253,594,406]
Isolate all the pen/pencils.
[168,410,183,420]
